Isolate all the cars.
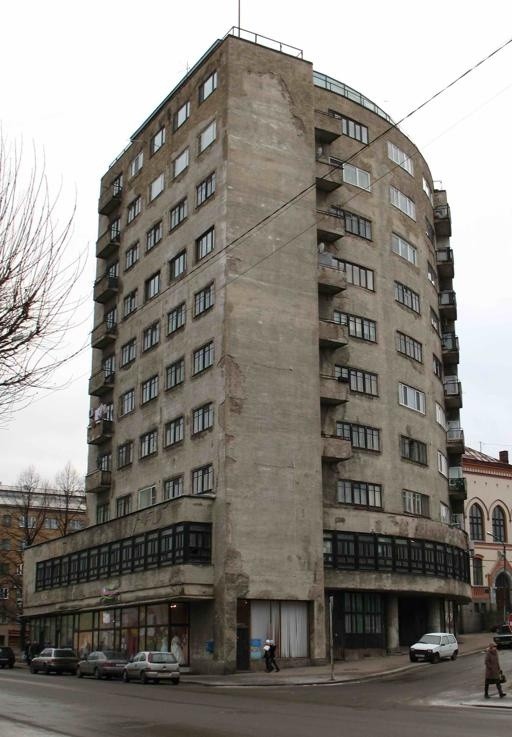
[122,650,181,684]
[30,646,79,675]
[493,622,512,649]
[76,650,126,680]
[0,646,15,668]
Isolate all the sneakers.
[485,694,506,698]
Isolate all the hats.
[489,642,498,649]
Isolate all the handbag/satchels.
[500,674,506,683]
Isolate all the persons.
[483,641,507,699]
[25,639,32,665]
[263,639,273,672]
[161,634,168,652]
[154,632,162,651]
[271,640,280,670]
[171,632,185,665]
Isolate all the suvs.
[409,631,459,663]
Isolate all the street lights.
[487,531,506,623]
